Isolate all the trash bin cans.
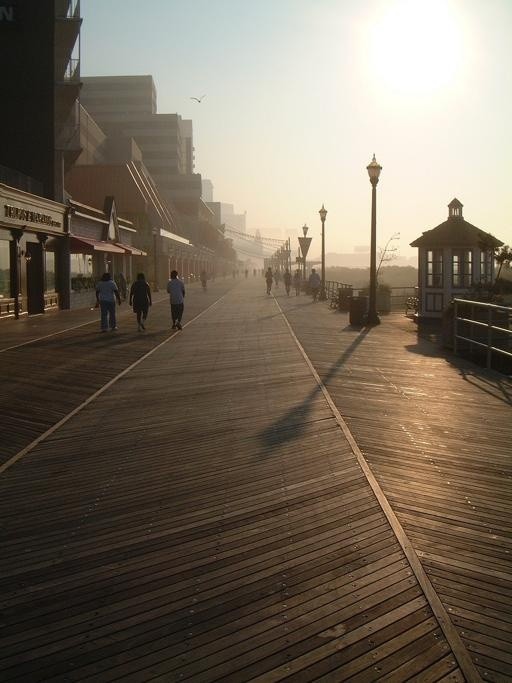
[338,288,354,312]
[347,296,368,325]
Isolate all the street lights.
[268,240,290,283]
[301,224,309,291]
[319,203,329,301]
[364,153,382,326]
[151,227,160,292]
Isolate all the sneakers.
[100,321,183,332]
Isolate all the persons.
[96,273,121,332]
[167,270,185,330]
[129,273,152,331]
[201,265,321,302]
